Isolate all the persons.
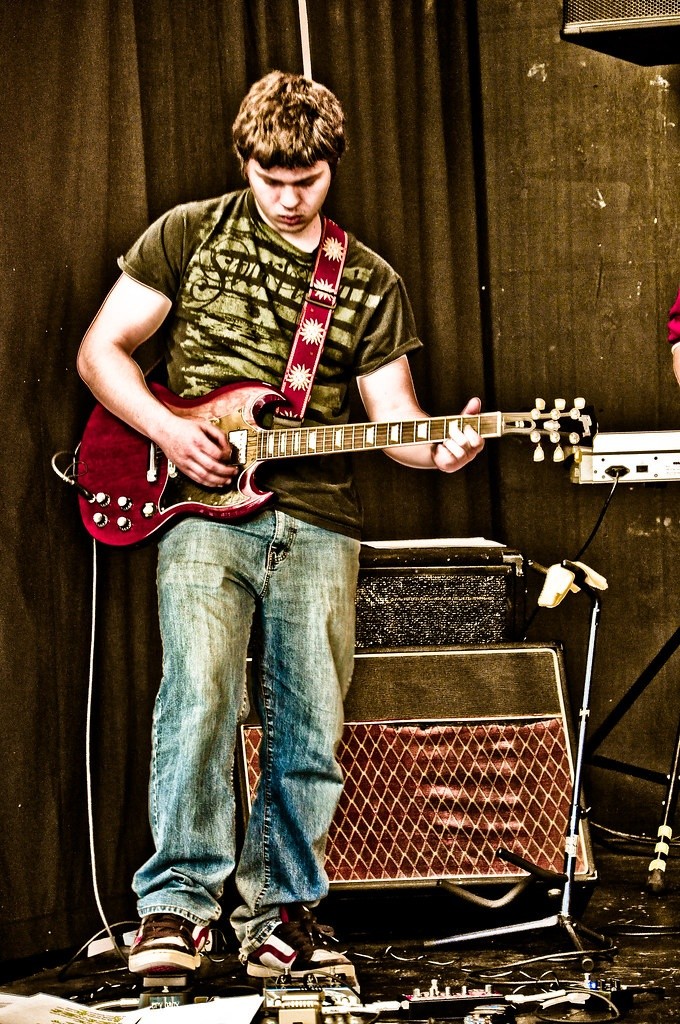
[74,71,486,981]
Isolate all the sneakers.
[127,913,214,975]
[245,920,351,978]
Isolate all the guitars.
[72,378,601,553]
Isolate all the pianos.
[563,427,679,487]
